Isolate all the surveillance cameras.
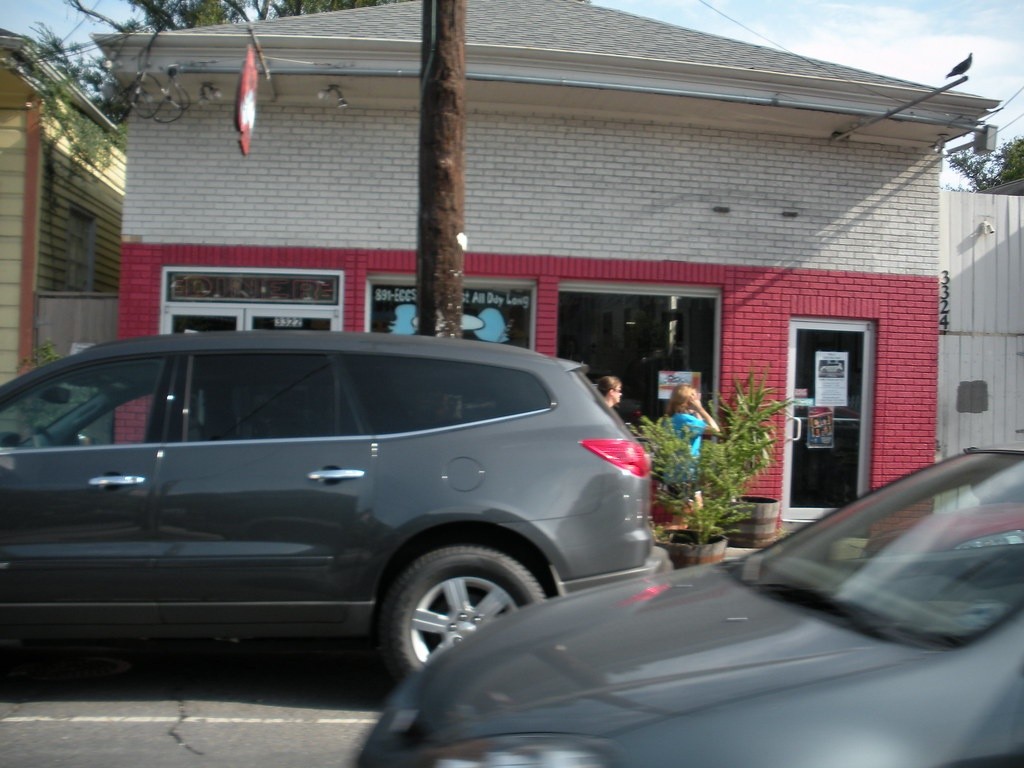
[986,225,995,233]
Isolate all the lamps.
[318,84,348,109]
[198,82,222,106]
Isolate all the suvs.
[0,330,671,680]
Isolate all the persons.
[664,384,720,530]
[813,417,830,436]
[597,376,622,408]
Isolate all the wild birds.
[945,51,972,77]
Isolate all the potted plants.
[709,365,802,548]
[630,413,755,568]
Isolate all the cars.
[360,440,1024,768]
